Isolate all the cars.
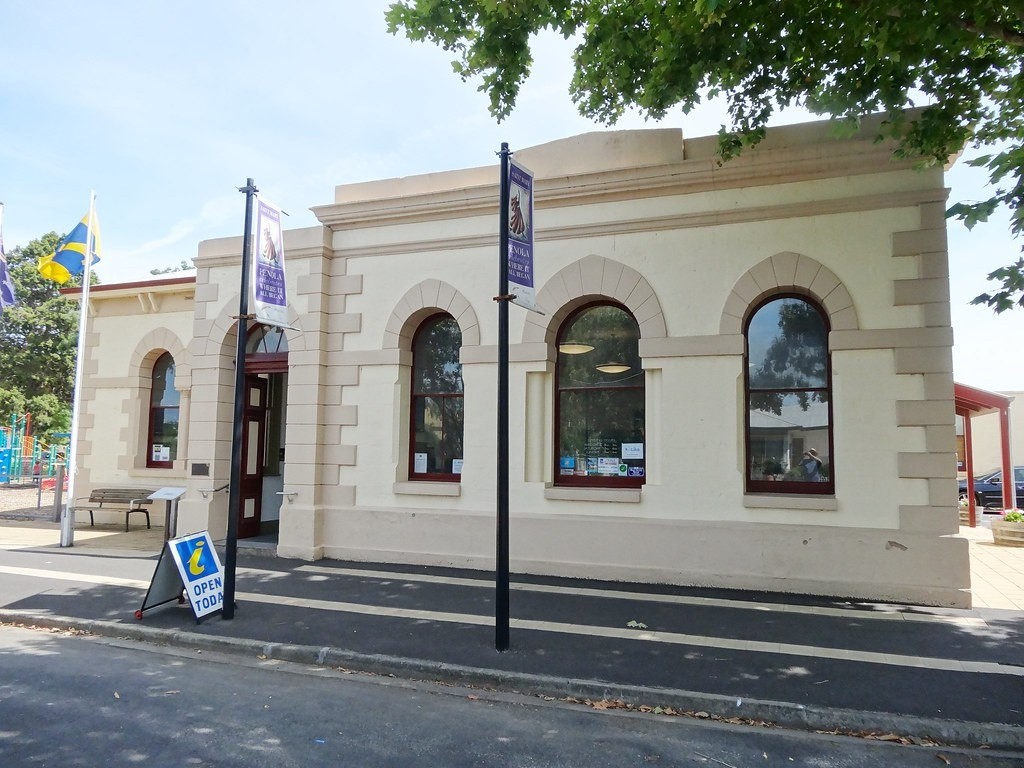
[958,465,1024,514]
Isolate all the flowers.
[958,498,968,506]
[998,508,1024,523]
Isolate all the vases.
[992,520,1024,547]
[959,506,985,526]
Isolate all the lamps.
[596,334,631,374]
[558,341,594,354]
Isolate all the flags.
[37,204,102,288]
[0,226,17,312]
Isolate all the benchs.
[72,488,156,532]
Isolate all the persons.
[33,460,40,483]
[795,447,823,482]
